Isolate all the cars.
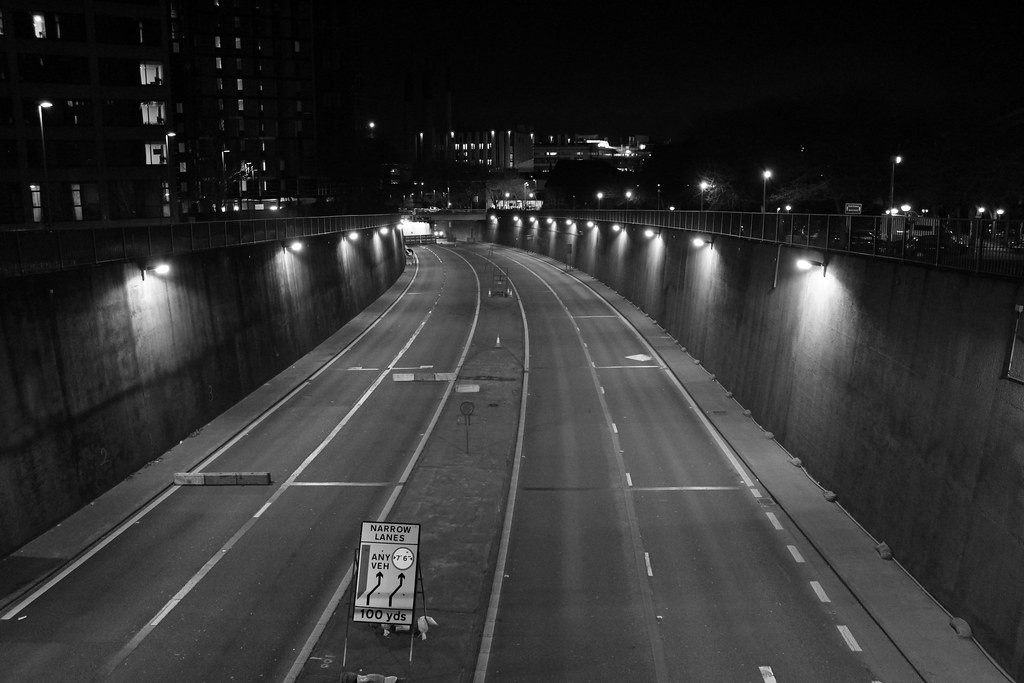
[434,229,444,238]
[987,226,1024,248]
[831,229,968,262]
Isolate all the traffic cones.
[505,286,513,297]
[486,288,491,297]
[493,333,504,349]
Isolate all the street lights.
[165,131,176,221]
[889,154,903,237]
[762,168,773,240]
[596,191,604,209]
[38,100,53,232]
[415,130,424,179]
[221,149,231,219]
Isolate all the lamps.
[692,234,716,249]
[586,221,599,228]
[612,223,626,231]
[138,260,172,281]
[644,228,661,241]
[566,219,577,225]
[280,240,304,255]
[342,230,360,243]
[793,252,833,277]
